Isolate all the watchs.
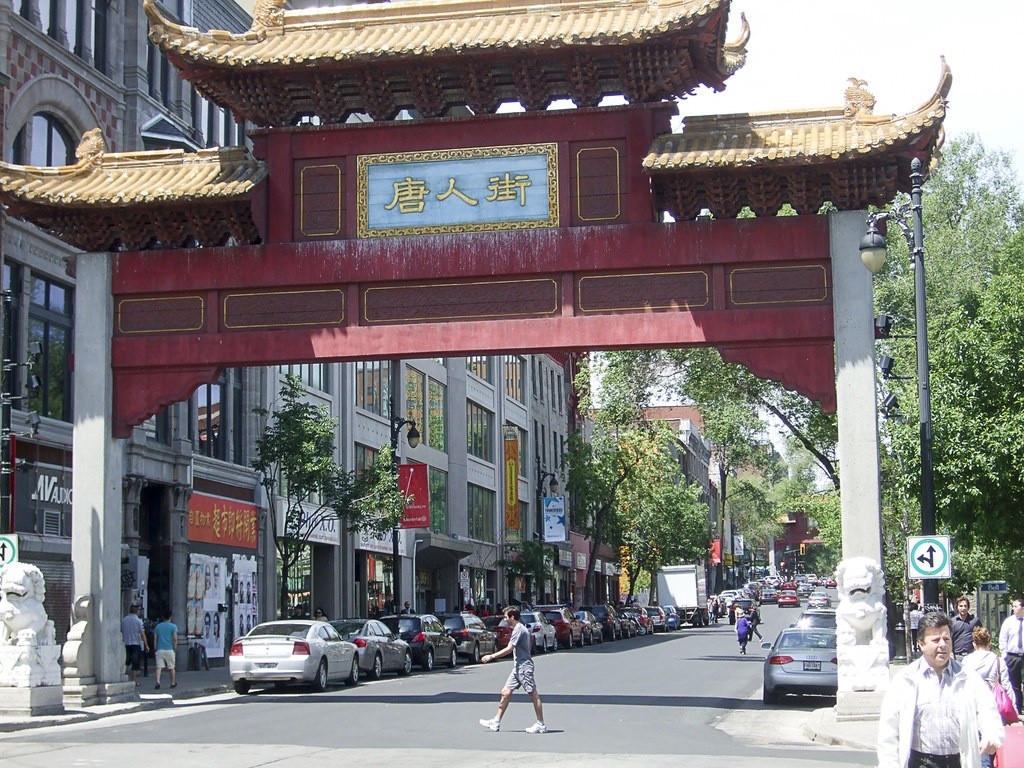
[489,655,493,660]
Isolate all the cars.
[709,568,837,631]
[760,627,838,704]
[480,615,541,657]
[328,619,413,680]
[519,611,558,654]
[229,619,360,695]
[573,610,603,646]
[612,604,681,639]
[776,589,800,608]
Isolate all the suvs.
[728,597,761,624]
[531,603,585,649]
[376,614,458,672]
[432,610,499,665]
[577,603,622,641]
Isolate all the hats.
[737,609,745,617]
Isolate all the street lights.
[389,394,421,615]
[859,156,939,614]
[535,456,560,605]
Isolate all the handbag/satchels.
[992,657,1019,723]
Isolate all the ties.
[1018,618,1023,649]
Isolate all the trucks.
[655,564,710,627]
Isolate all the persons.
[154,612,178,689]
[311,609,328,622]
[949,597,984,662]
[465,603,502,617]
[961,625,1018,768]
[291,605,308,620]
[998,599,1024,717]
[877,612,1006,768]
[479,607,547,735]
[707,598,718,625]
[909,604,922,652]
[401,601,416,614]
[735,606,764,655]
[121,605,149,686]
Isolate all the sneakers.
[526,722,547,733]
[479,719,500,732]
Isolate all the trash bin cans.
[188,643,203,671]
[894,622,906,660]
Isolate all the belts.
[1007,652,1024,657]
[955,652,967,656]
